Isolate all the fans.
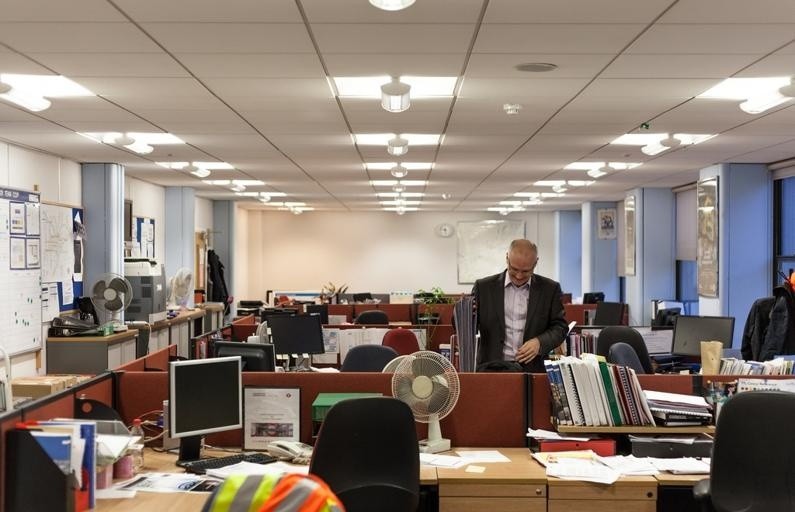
[167,266,194,312]
[88,273,135,332]
[391,350,461,453]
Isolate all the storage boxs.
[11,377,79,398]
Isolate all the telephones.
[267,440,314,464]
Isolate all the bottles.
[128,419,145,468]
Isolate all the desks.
[534,440,710,512]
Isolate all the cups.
[113,455,134,478]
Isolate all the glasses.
[506,255,538,273]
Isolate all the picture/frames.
[695,175,719,298]
[623,197,636,277]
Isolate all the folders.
[47,317,98,337]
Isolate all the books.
[3,418,99,511]
[542,330,794,426]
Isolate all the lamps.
[365,78,416,218]
[77,437,548,511]
[497,84,795,215]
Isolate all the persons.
[450,238,569,374]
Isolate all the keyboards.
[185,452,278,475]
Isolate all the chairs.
[383,329,420,355]
[341,345,398,373]
[609,342,645,374]
[354,310,391,323]
[596,326,654,373]
[692,391,794,512]
[309,396,420,512]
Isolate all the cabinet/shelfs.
[46,301,224,374]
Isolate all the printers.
[122,257,168,325]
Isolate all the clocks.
[438,224,454,239]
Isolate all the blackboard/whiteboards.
[456,221,526,284]
[1,186,43,360]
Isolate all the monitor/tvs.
[214,341,275,371]
[671,315,735,357]
[594,301,624,325]
[261,309,298,328]
[169,356,244,466]
[583,292,605,303]
[307,305,328,324]
[271,315,325,371]
